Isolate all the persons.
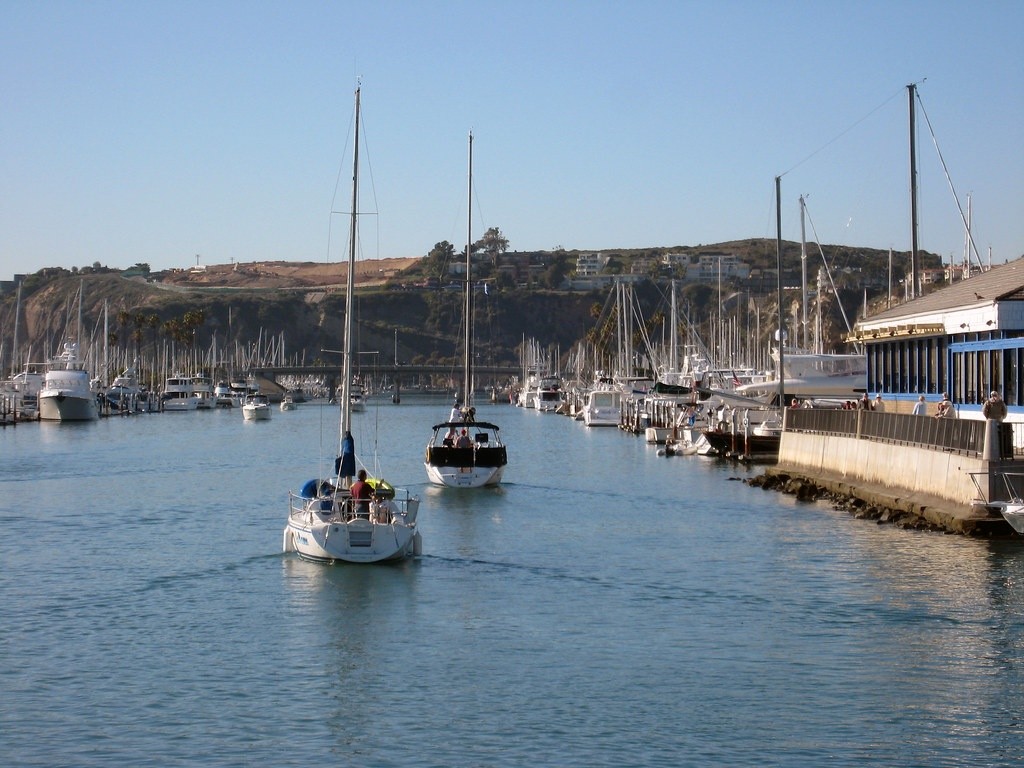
[842,400,856,410]
[856,392,874,411]
[449,403,462,439]
[873,394,885,411]
[982,391,1007,421]
[912,396,927,415]
[937,392,956,418]
[456,430,470,448]
[351,470,375,519]
[791,399,801,408]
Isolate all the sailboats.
[0,276,368,428]
[484,79,868,461]
[283,77,425,564]
[422,127,508,491]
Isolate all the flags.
[733,371,742,386]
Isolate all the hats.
[991,391,998,395]
[863,393,868,397]
[461,429,466,434]
[877,393,881,397]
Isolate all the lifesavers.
[718,421,729,433]
[425,447,431,464]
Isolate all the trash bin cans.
[998,423,1014,459]
